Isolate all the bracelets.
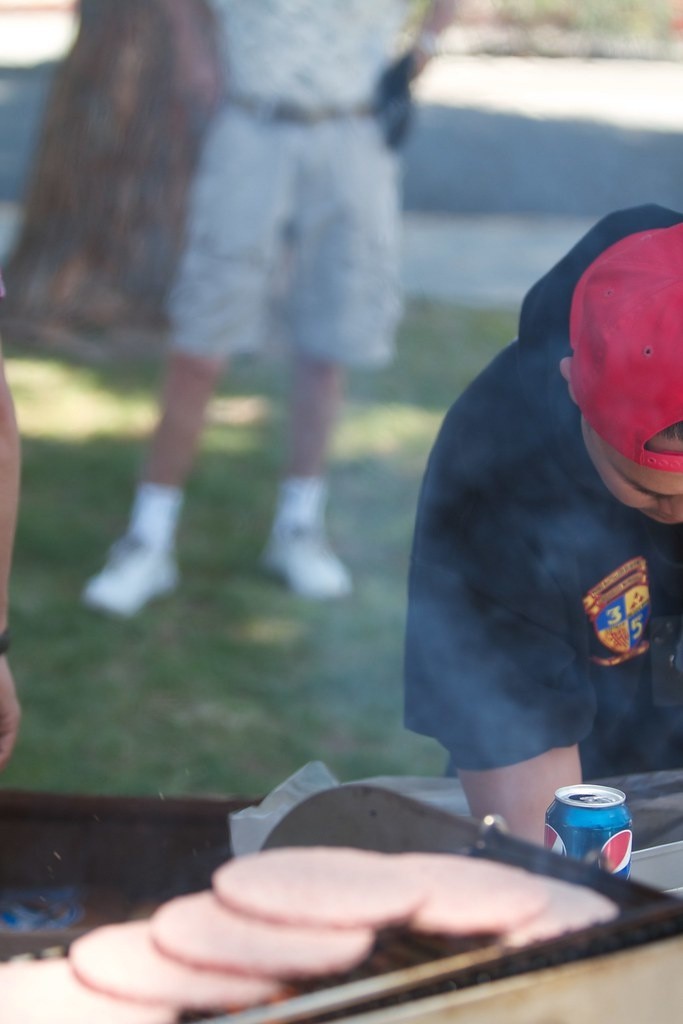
[0,628,11,654]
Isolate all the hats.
[570,222,683,472]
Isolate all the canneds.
[543,783,632,883]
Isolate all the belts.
[233,91,381,128]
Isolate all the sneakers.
[82,534,180,617]
[262,526,351,599]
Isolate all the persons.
[403,203,683,850]
[0,277,21,772]
[79,0,459,619]
